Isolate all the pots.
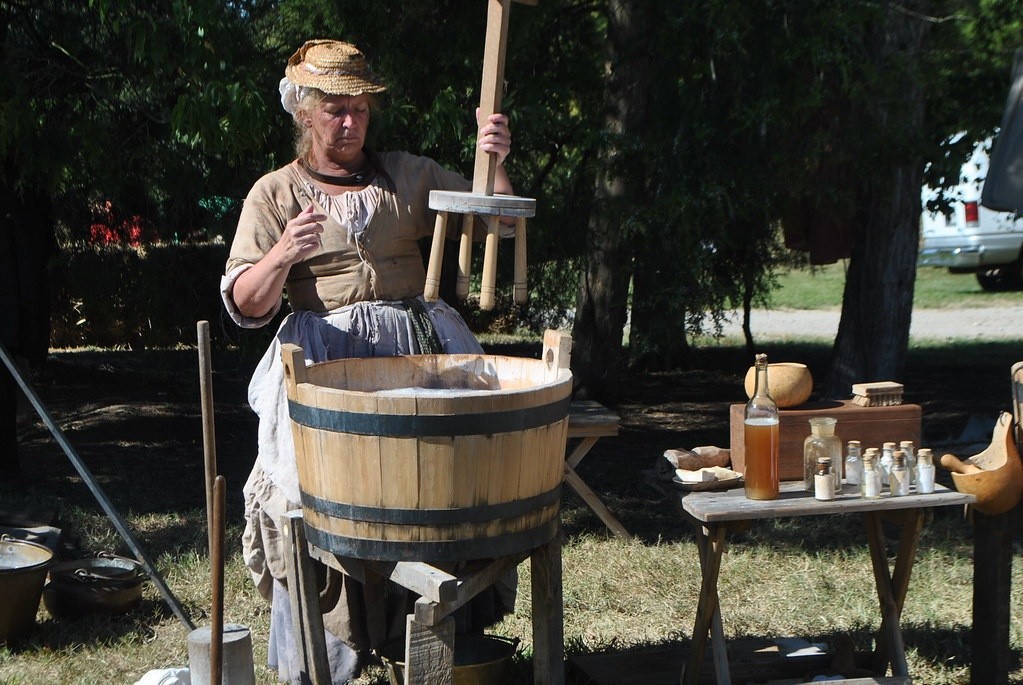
[380,637,514,685]
[43,557,144,642]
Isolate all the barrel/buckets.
[0,533,54,648]
[281,328,573,562]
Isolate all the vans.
[922,126,1023,291]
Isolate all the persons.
[218,37,522,678]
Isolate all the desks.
[669,479,979,685]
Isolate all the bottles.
[860,448,883,498]
[889,451,910,495]
[846,440,863,485]
[814,457,836,501]
[917,449,935,494]
[803,417,842,492]
[743,353,781,500]
[899,441,916,485]
[879,442,896,484]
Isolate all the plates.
[673,475,744,492]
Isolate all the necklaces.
[296,142,376,187]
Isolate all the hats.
[284,38,391,94]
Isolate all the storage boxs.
[377,633,521,685]
[729,399,923,481]
[50,550,144,619]
[280,327,576,563]
[950,411,1022,516]
[744,362,813,409]
[0,534,54,650]
